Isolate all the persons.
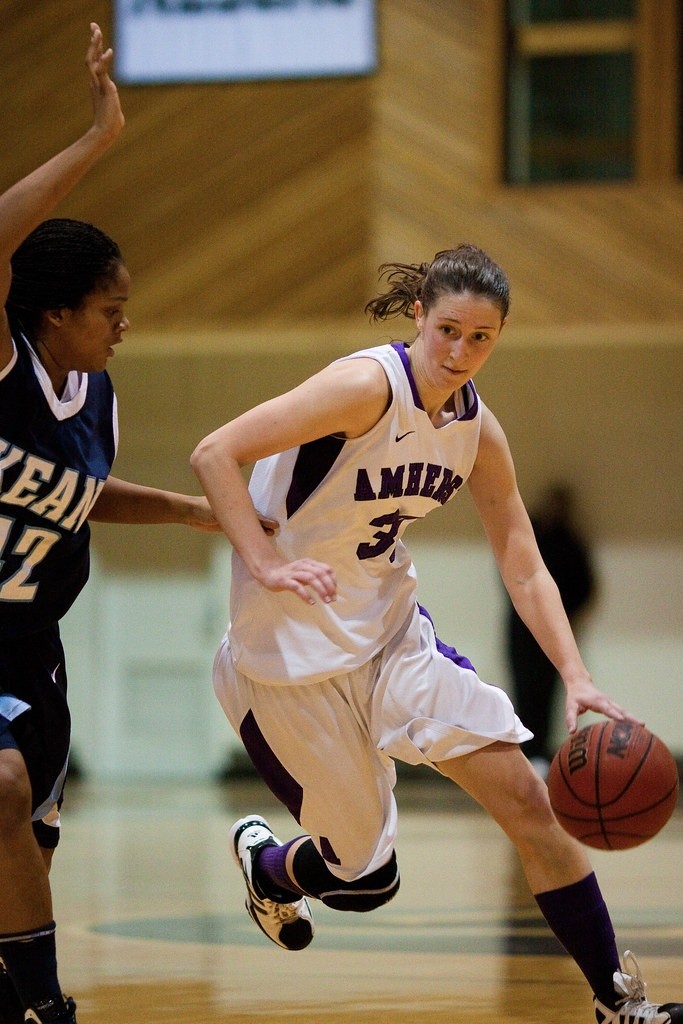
[189,243,683,1024]
[0,22,280,1024]
[505,482,598,764]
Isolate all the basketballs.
[545,717,681,853]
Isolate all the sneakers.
[592,992,683,1024]
[227,813,317,954]
[21,993,78,1024]
